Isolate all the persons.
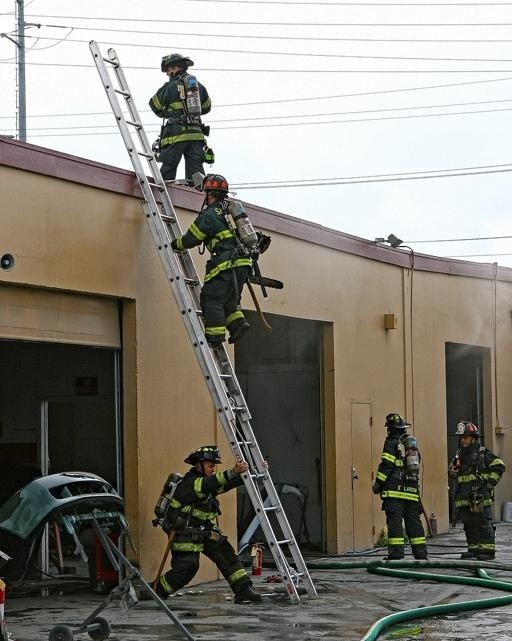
[132,443,269,604]
[448,421,506,560]
[148,53,211,188]
[170,174,252,346]
[373,413,428,560]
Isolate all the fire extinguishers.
[250,543,265,575]
[429,512,438,535]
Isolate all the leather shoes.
[234,585,261,601]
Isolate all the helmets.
[456,420,481,436]
[385,412,412,429]
[184,445,222,465]
[161,53,194,71]
[201,173,228,192]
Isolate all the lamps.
[375,234,402,248]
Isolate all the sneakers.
[228,321,251,343]
[478,553,496,559]
[204,333,226,342]
[461,551,478,558]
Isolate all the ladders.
[90,40,318,603]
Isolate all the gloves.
[372,483,382,494]
